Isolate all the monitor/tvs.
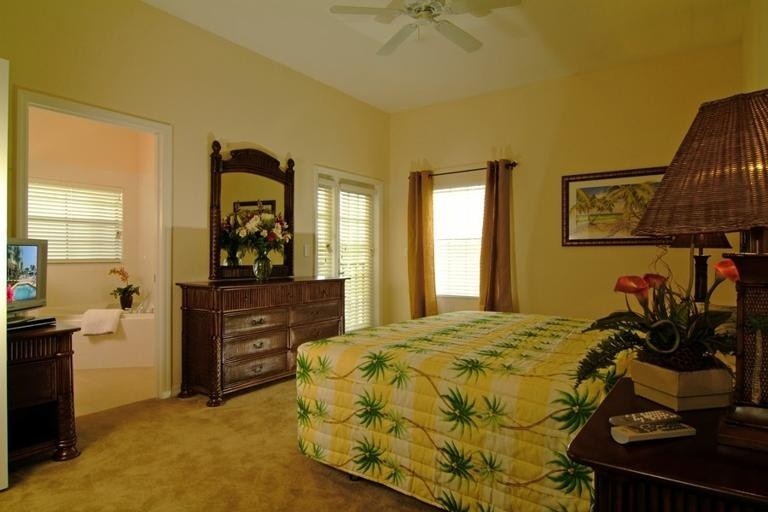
[7,237,47,325]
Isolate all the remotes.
[608,410,683,426]
[610,420,697,444]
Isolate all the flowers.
[572,250,740,388]
[106,265,141,300]
[230,199,293,280]
[220,213,249,253]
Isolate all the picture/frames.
[561,164,683,248]
[233,200,277,218]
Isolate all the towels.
[80,308,123,337]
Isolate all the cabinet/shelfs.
[174,272,352,408]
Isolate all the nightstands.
[563,375,768,511]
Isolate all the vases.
[226,248,239,266]
[252,244,275,283]
[630,353,735,412]
[119,294,134,311]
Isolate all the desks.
[7,314,81,468]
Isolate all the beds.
[294,308,736,511]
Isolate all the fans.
[325,0,529,58]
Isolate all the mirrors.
[208,139,295,283]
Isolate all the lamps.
[628,85,768,427]
[669,229,733,307]
